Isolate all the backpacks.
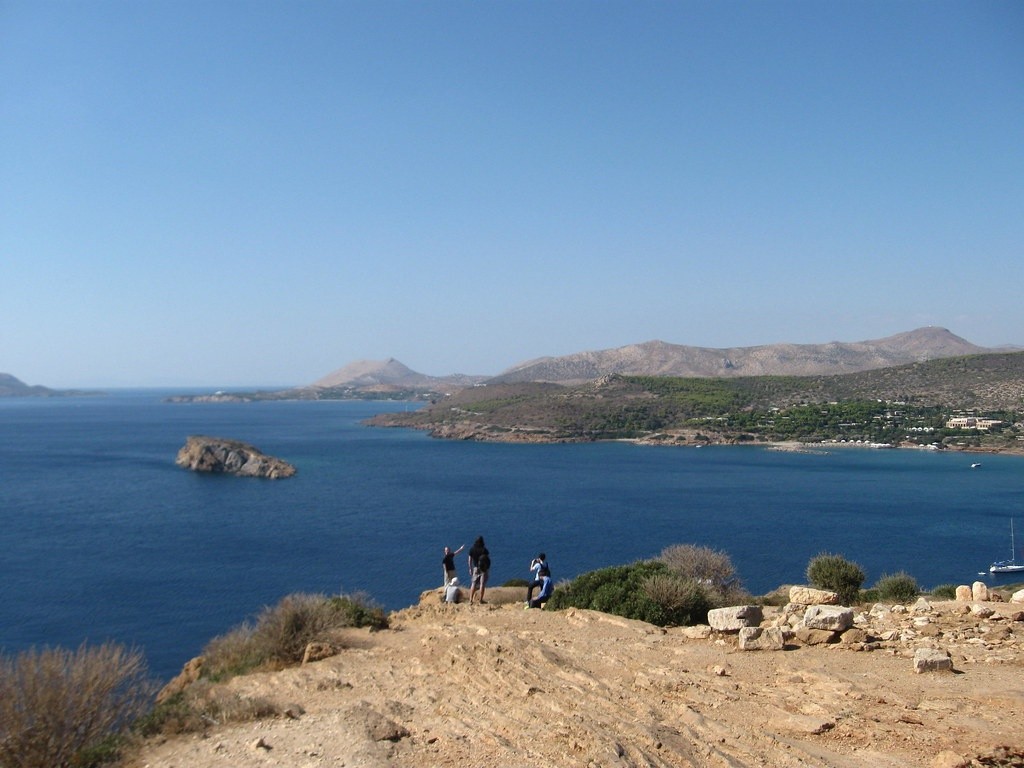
[477,554,490,572]
[539,561,550,577]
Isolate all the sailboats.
[990,517,1023,572]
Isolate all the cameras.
[534,560,539,562]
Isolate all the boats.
[971,462,981,467]
[978,571,986,575]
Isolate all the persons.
[530,570,553,608]
[443,577,463,604]
[442,543,466,586]
[527,552,550,602]
[468,536,491,605]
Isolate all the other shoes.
[469,602,472,605]
[479,601,488,604]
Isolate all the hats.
[449,577,458,585]
